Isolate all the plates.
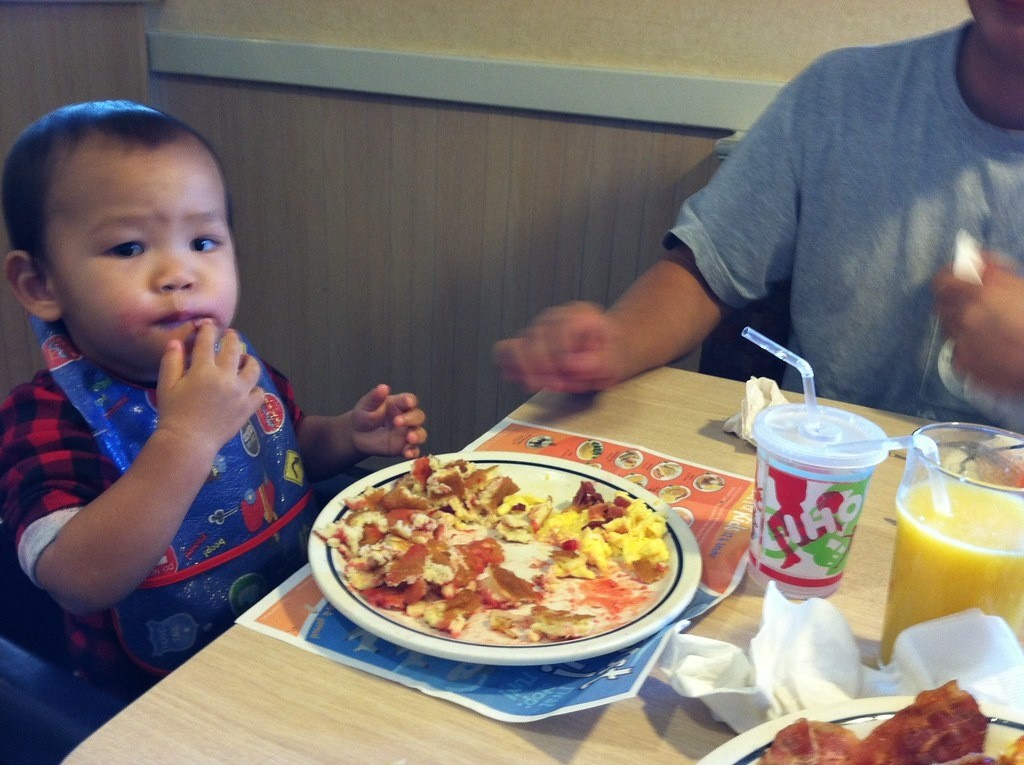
[308,451,702,665]
[697,696,1022,765]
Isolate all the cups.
[878,422,1024,670]
[747,402,890,600]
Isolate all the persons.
[492,0,1024,435]
[0,101,428,692]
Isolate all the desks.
[45,366,1024,764]
